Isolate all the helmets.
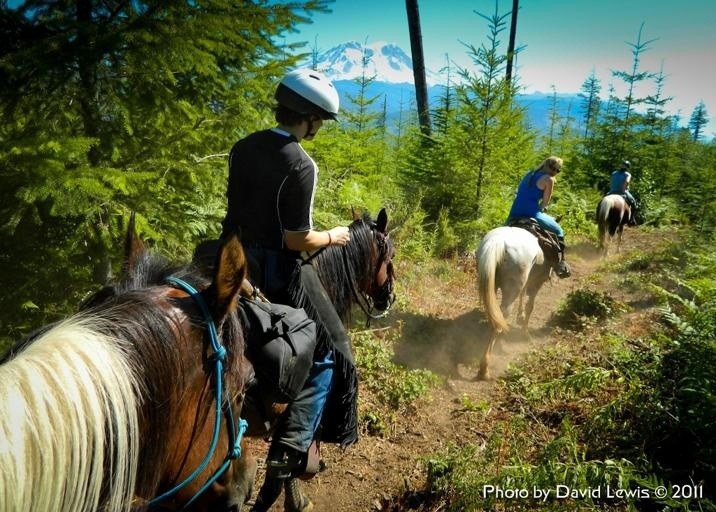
[276,68,338,121]
[622,160,632,168]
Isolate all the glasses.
[548,165,562,175]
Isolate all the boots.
[557,235,571,276]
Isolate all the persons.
[606,160,639,225]
[504,156,571,279]
[212,69,357,481]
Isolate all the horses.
[595,193,632,258]
[0,204,398,511]
[473,212,566,381]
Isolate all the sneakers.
[268,445,326,478]
